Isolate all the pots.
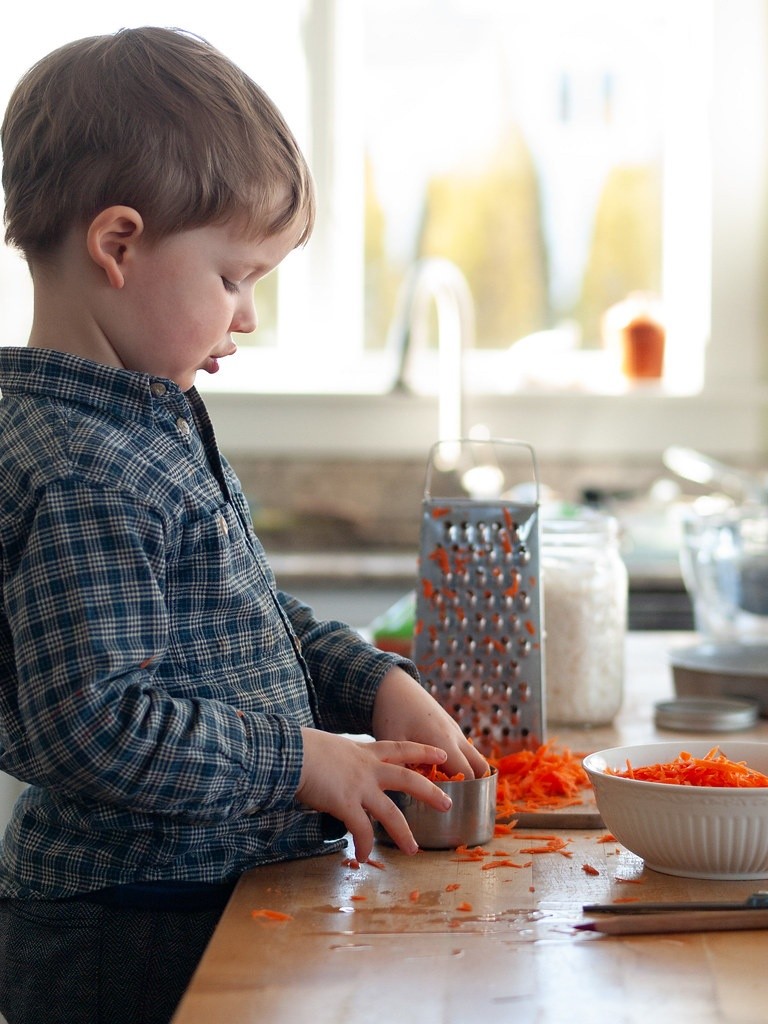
[318,765,498,847]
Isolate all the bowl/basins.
[582,740,768,880]
[673,646,768,714]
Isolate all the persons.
[0,26,491,1023]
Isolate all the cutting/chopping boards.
[495,789,606,828]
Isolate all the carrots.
[252,501,645,920]
[602,744,768,788]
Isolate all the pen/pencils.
[574,910,768,936]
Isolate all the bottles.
[539,513,627,727]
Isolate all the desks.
[169,629,768,1024]
